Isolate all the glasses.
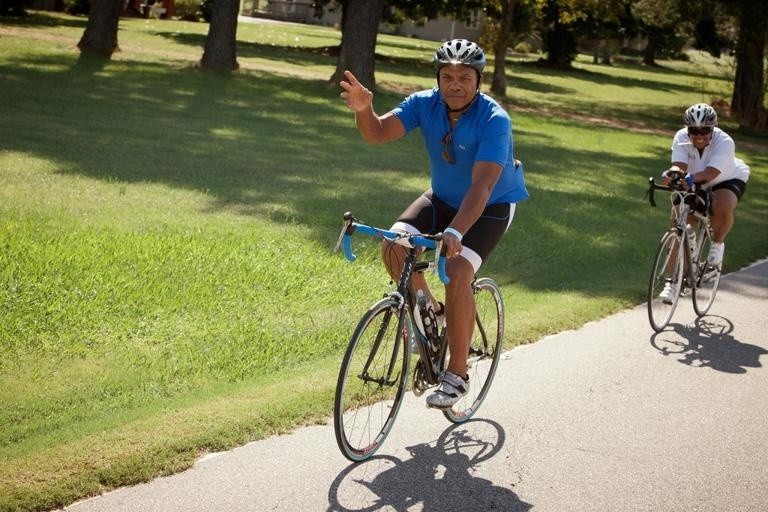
[688,127,711,135]
[440,133,454,163]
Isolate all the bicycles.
[643,173,726,330]
[333,211,506,460]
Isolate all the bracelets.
[443,226,463,244]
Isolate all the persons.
[339,36,531,411]
[657,99,752,309]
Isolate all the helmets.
[685,104,717,128]
[434,39,485,71]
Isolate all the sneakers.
[659,283,674,301]
[708,242,724,266]
[412,301,445,354]
[427,371,470,407]
